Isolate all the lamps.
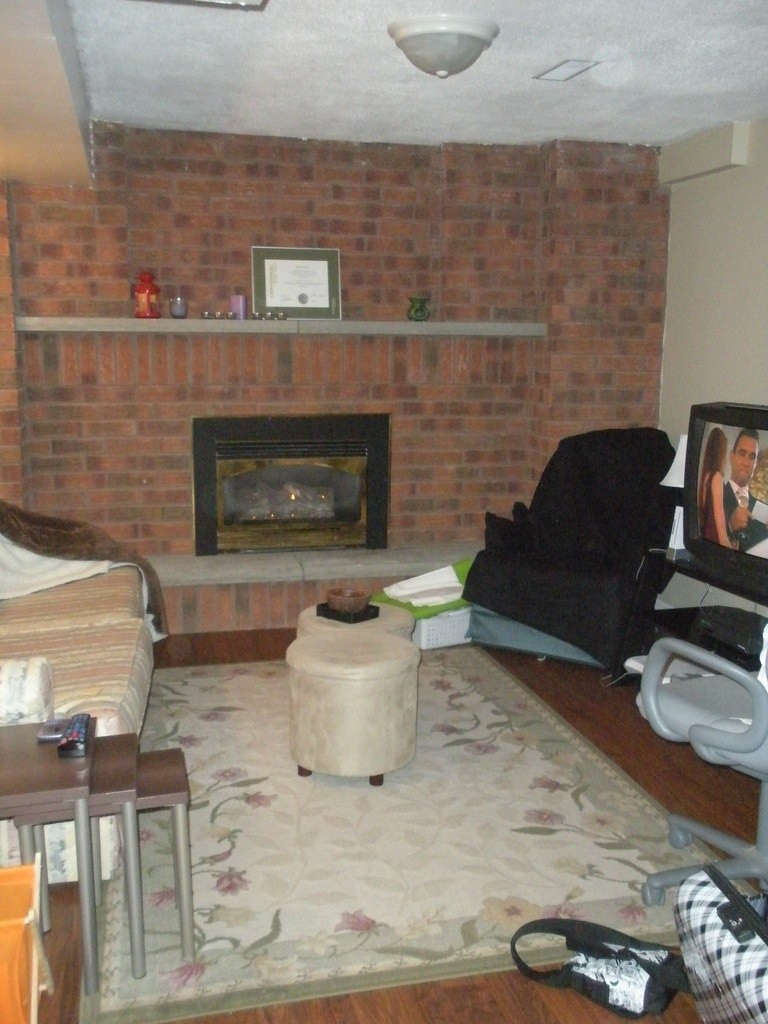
[657,433,688,487]
[387,14,501,78]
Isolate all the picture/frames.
[250,244,343,321]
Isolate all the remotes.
[55,713,91,757]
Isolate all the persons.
[698,427,768,550]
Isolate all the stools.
[285,601,423,785]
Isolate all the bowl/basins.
[326,588,372,614]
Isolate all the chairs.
[625,634,768,918]
[464,425,675,670]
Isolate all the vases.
[406,296,430,321]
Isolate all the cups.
[168,298,189,318]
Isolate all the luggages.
[674,865,768,1024]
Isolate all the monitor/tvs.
[681,400,768,588]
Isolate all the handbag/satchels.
[511,919,691,1018]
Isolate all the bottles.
[133,273,161,318]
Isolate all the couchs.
[0,502,155,733]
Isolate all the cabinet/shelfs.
[0,717,195,995]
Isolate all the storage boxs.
[379,605,471,650]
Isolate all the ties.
[737,487,745,507]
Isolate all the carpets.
[78,647,761,1024]
